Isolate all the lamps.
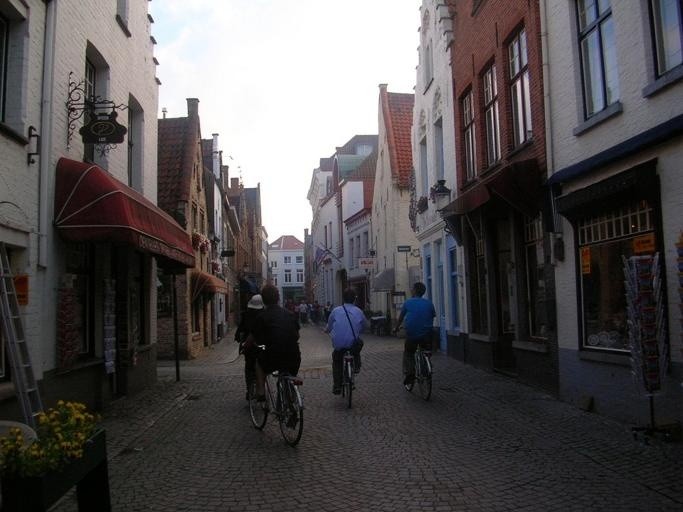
[432,179,453,234]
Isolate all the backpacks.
[272,324,302,377]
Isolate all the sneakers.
[286,410,298,427]
[403,374,416,385]
[254,385,266,403]
[332,384,342,394]
[353,366,361,374]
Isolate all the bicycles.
[342,350,356,411]
[245,364,305,448]
[393,323,434,404]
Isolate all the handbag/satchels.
[352,336,364,353]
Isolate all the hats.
[246,294,265,310]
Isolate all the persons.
[235,293,266,401]
[242,285,301,401]
[281,299,334,326]
[323,288,367,394]
[392,282,437,384]
